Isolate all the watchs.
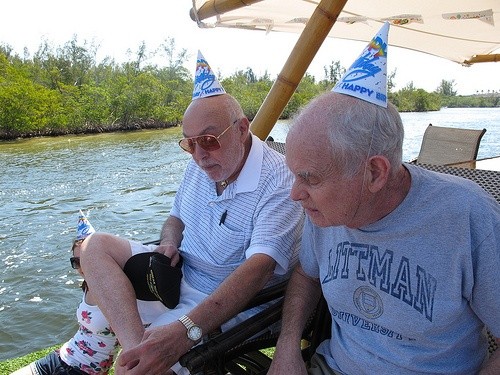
[176,315,203,345]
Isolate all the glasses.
[178,119,237,155]
[69,257,82,269]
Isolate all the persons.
[7,239,121,375]
[268,91,500,375]
[80,94,307,375]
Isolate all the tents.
[190,0,500,141]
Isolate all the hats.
[122,251,184,310]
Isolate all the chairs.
[405,124,488,170]
[178,138,500,375]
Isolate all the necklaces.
[217,181,230,189]
